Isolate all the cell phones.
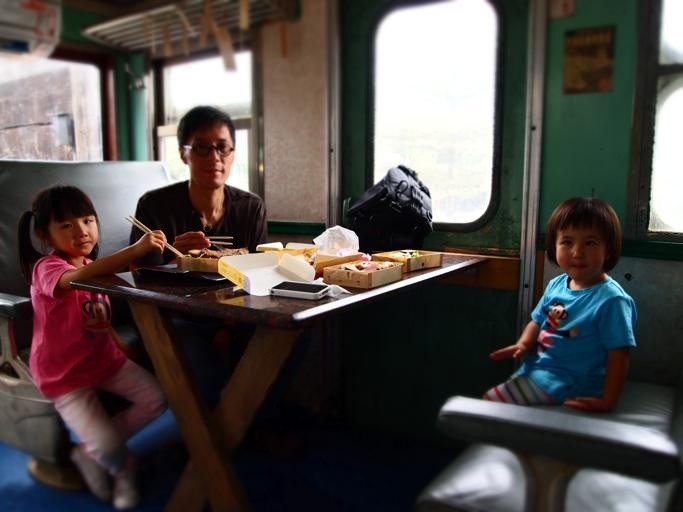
[269,280,330,300]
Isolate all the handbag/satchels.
[346,165,433,248]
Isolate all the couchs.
[415,361,683,511]
[0,160,174,491]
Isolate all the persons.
[17,184,166,508]
[130,105,267,264]
[483,196,638,412]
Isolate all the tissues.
[313,224,363,280]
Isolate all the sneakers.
[70,444,111,502]
[112,452,139,510]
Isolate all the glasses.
[183,141,235,157]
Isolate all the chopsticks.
[124,215,186,258]
[176,236,234,244]
